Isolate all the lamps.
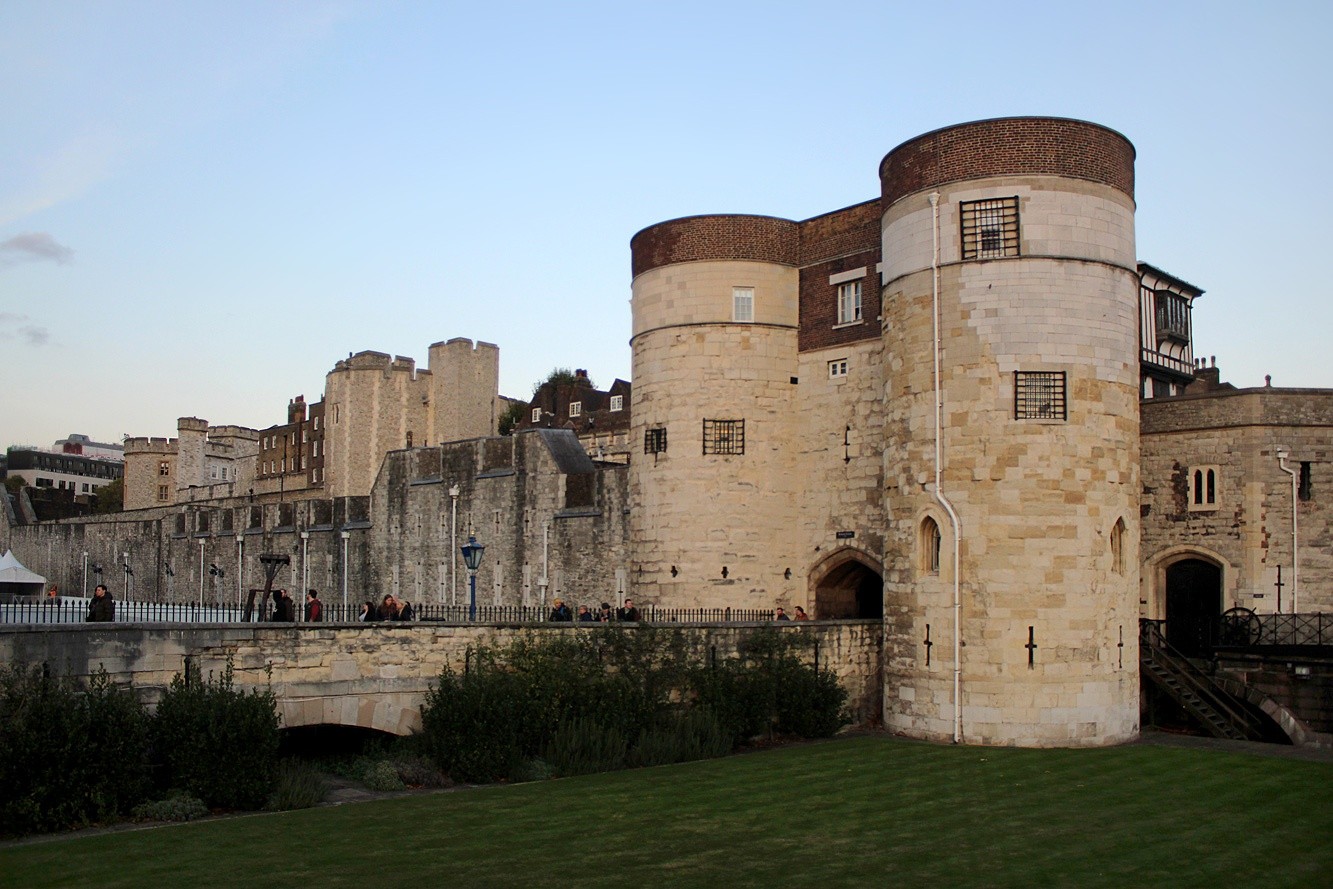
[209,564,222,577]
[92,565,102,573]
[123,564,134,576]
[165,562,174,576]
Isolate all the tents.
[0,549,47,603]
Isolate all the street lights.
[460,537,486,621]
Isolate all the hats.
[554,598,561,604]
[602,603,611,609]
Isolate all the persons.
[272,589,412,622]
[775,606,810,620]
[548,598,641,623]
[89,585,115,622]
